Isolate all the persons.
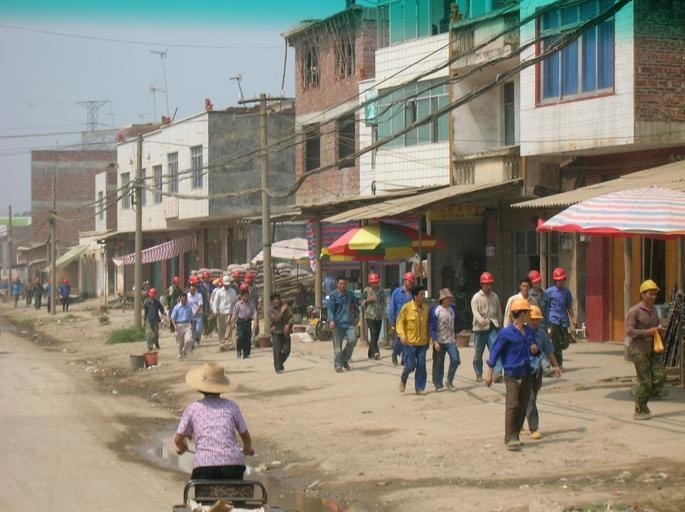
[471,272,503,383]
[173,362,252,501]
[267,293,294,373]
[11,268,77,315]
[504,268,578,377]
[322,269,358,373]
[427,287,463,392]
[517,304,564,439]
[389,271,415,366]
[295,283,307,320]
[144,269,258,359]
[484,294,540,451]
[359,271,386,360]
[622,278,672,422]
[396,285,430,393]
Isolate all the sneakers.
[237,350,249,359]
[506,428,541,448]
[633,411,649,421]
[336,362,349,372]
[369,351,404,366]
[399,380,456,395]
[543,367,553,376]
[476,373,504,382]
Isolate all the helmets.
[173,275,180,283]
[480,272,495,284]
[148,287,158,296]
[369,273,380,284]
[404,272,413,282]
[510,298,543,319]
[528,270,540,283]
[189,271,253,291]
[551,267,566,281]
[35,277,69,286]
[639,280,660,293]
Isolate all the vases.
[129,353,144,369]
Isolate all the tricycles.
[175,445,274,511]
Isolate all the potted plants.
[144,330,158,366]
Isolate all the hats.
[185,360,239,395]
[438,287,453,301]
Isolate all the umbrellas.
[534,183,684,278]
[324,220,445,260]
[249,233,313,314]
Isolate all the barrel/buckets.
[130,355,144,370]
[259,336,270,348]
[143,352,159,368]
[457,334,470,347]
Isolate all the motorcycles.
[305,305,329,342]
[117,280,153,309]
